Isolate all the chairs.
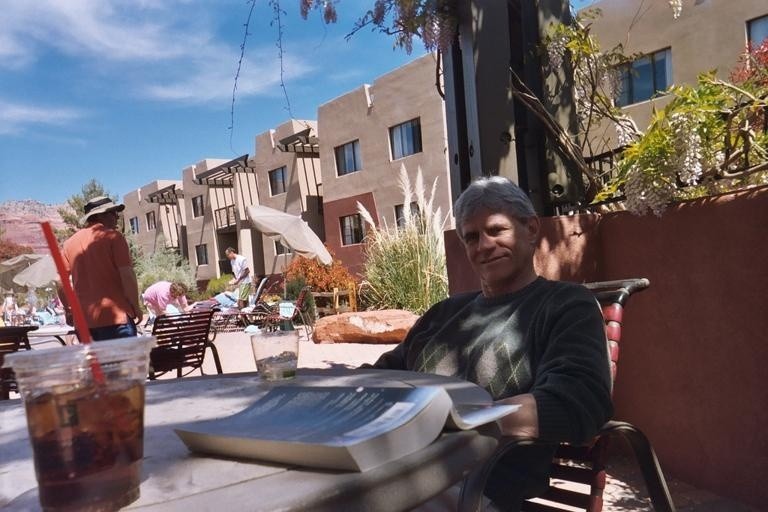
[482,276,678,510]
[2,277,315,400]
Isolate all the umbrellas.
[244,204,333,275]
[11,253,62,290]
[0,251,49,294]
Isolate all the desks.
[4,370,495,509]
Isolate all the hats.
[82,196,126,224]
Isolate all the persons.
[55,195,144,344]
[225,246,253,329]
[356,174,615,511]
[0,283,66,330]
[140,280,194,348]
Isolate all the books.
[171,384,525,474]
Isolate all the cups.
[248,330,300,384]
[1,336,158,512]
